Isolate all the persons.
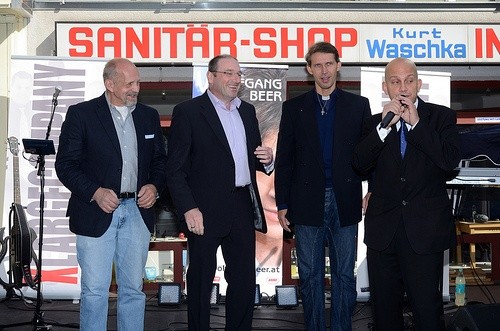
[185,67,287,297]
[274,42,372,331]
[166,54,275,331]
[351,58,459,331]
[55,57,168,331]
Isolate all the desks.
[455,219,500,282]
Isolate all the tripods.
[0,139,80,331]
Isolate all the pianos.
[439,165,500,304]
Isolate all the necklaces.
[317,94,327,115]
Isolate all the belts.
[119,191,135,199]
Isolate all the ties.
[397,119,409,160]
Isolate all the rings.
[191,227,194,228]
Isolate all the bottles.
[455,268,466,307]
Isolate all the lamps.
[275,286,299,310]
[253,284,261,309]
[158,283,181,309]
[209,283,219,309]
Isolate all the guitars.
[3,136,33,289]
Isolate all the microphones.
[51,85,62,102]
[380,94,406,128]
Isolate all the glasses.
[210,71,243,78]
